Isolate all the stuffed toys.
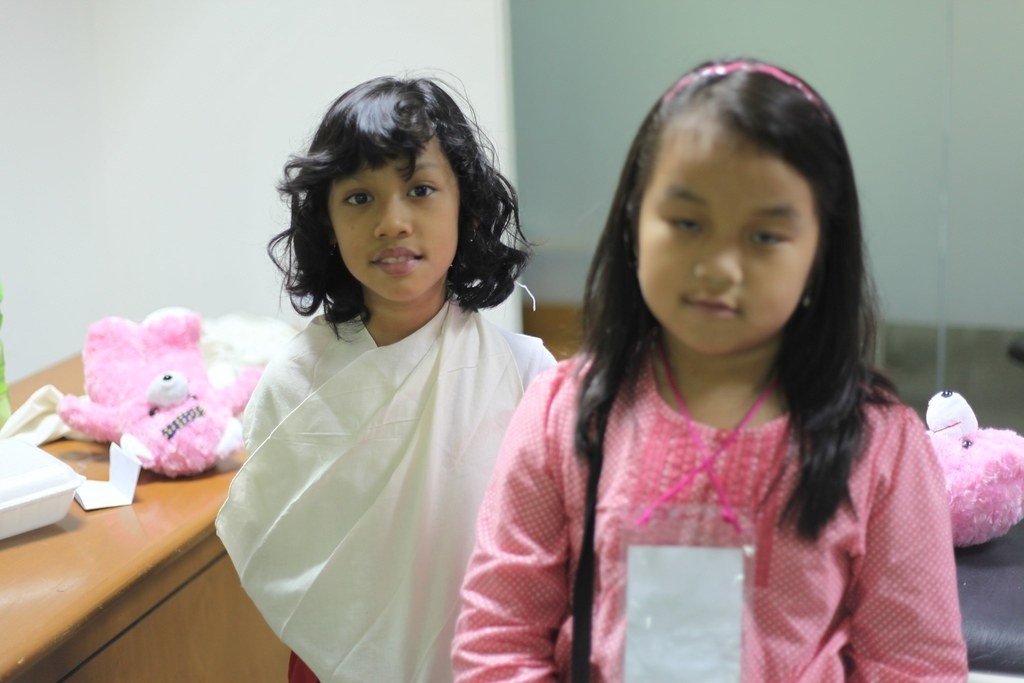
[58,305,263,477]
[924,389,1024,545]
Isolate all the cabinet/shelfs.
[2,351,289,683]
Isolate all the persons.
[213,76,556,683]
[451,56,967,683]
